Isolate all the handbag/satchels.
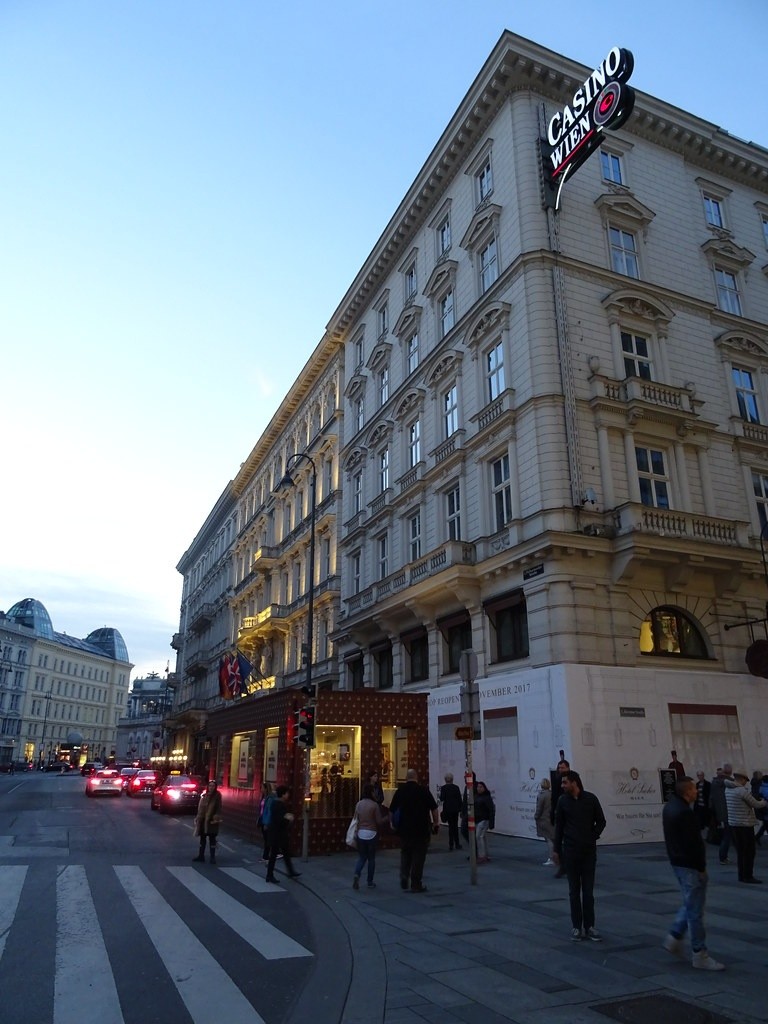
[346,804,361,850]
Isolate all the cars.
[80,762,106,777]
[119,767,141,788]
[40,761,74,773]
[127,770,163,799]
[1,760,33,773]
[150,775,208,815]
[86,770,123,798]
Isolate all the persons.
[535,759,570,866]
[265,785,302,883]
[361,770,385,805]
[388,768,439,893]
[191,780,221,864]
[259,782,283,860]
[694,763,768,865]
[178,760,210,783]
[440,771,495,863]
[663,778,722,970]
[551,771,606,941]
[725,769,766,883]
[351,784,381,889]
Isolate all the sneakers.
[662,936,686,963]
[690,950,726,971]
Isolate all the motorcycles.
[7,763,15,776]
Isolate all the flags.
[218,647,253,700]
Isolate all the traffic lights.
[292,708,306,747]
[301,684,316,700]
[298,705,316,749]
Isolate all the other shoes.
[259,859,269,863]
[719,857,734,865]
[542,858,557,867]
[585,928,602,942]
[411,884,426,892]
[744,877,763,884]
[276,854,283,859]
[368,882,377,888]
[570,928,582,941]
[475,856,487,865]
[193,854,205,862]
[400,873,409,889]
[353,875,360,889]
[209,856,216,863]
[555,870,570,879]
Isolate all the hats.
[733,767,751,782]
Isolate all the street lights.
[280,453,318,857]
[38,691,52,767]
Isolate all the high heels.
[265,874,280,883]
[288,871,303,880]
